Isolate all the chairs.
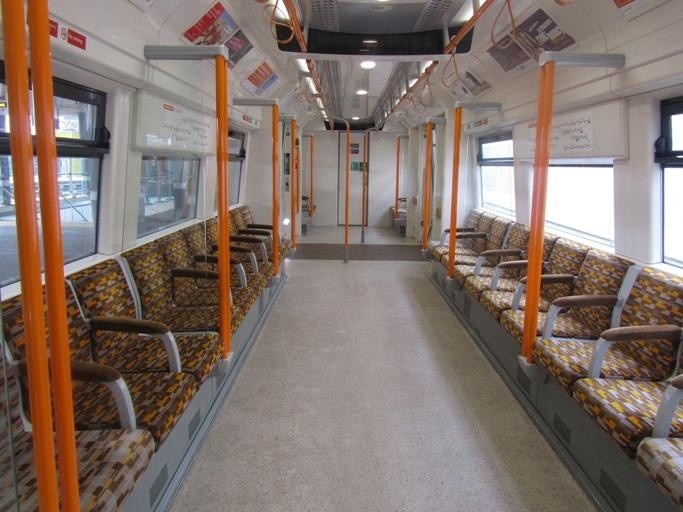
[302,196,316,235]
[478,237,682,511]
[429,209,557,345]
[1,268,233,512]
[393,198,407,236]
[94,205,292,378]
